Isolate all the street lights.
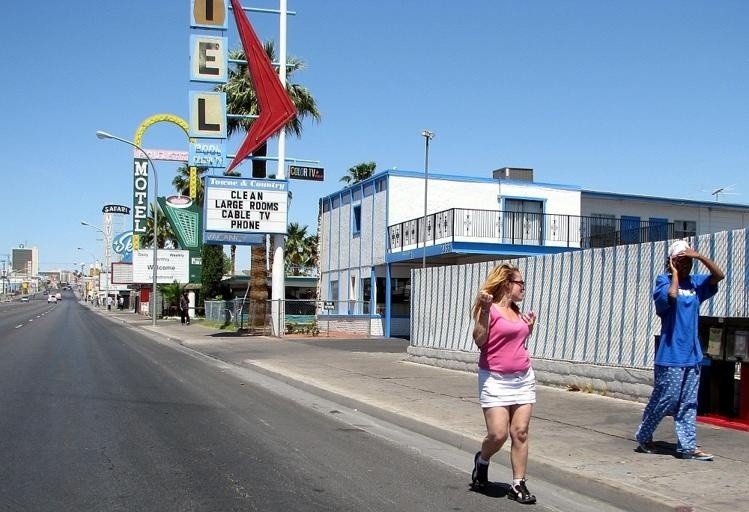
[94,129,158,326]
[78,247,103,272]
[421,127,434,268]
[80,220,110,311]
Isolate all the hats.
[668,241,690,261]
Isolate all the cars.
[20,284,72,304]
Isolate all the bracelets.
[481,309,489,314]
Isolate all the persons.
[469,262,537,504]
[95,296,100,307]
[118,295,125,311]
[179,292,191,326]
[633,239,725,460]
[106,295,112,310]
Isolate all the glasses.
[510,280,524,285]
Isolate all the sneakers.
[635,432,659,454]
[681,450,714,460]
[507,477,536,503]
[472,451,489,488]
